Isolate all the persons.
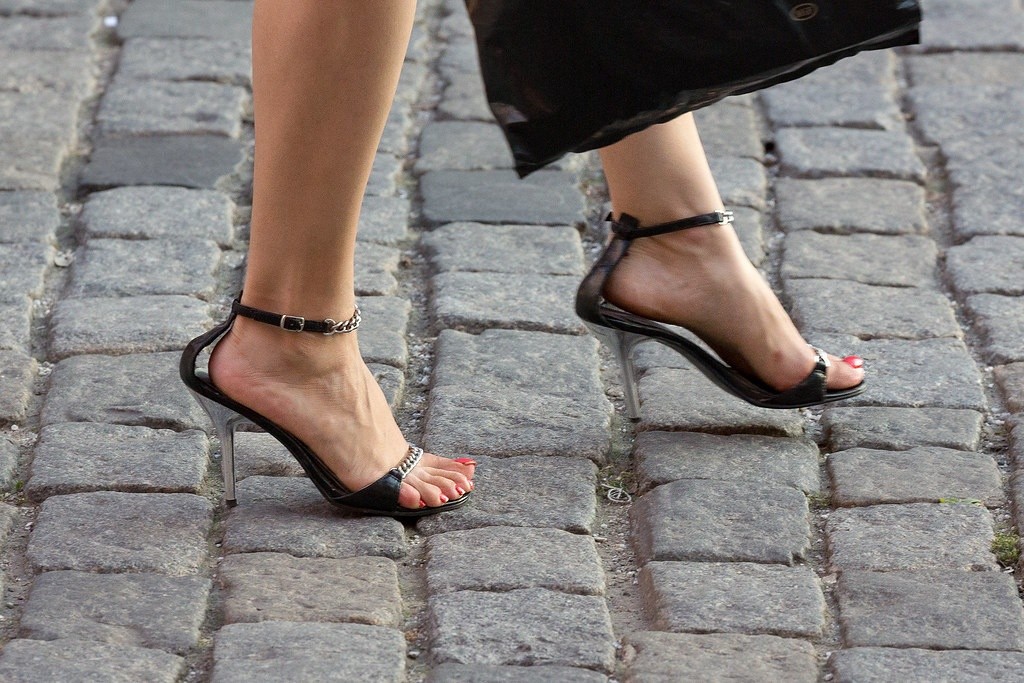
[176,0,870,514]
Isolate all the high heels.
[178,287,472,518]
[574,213,867,423]
